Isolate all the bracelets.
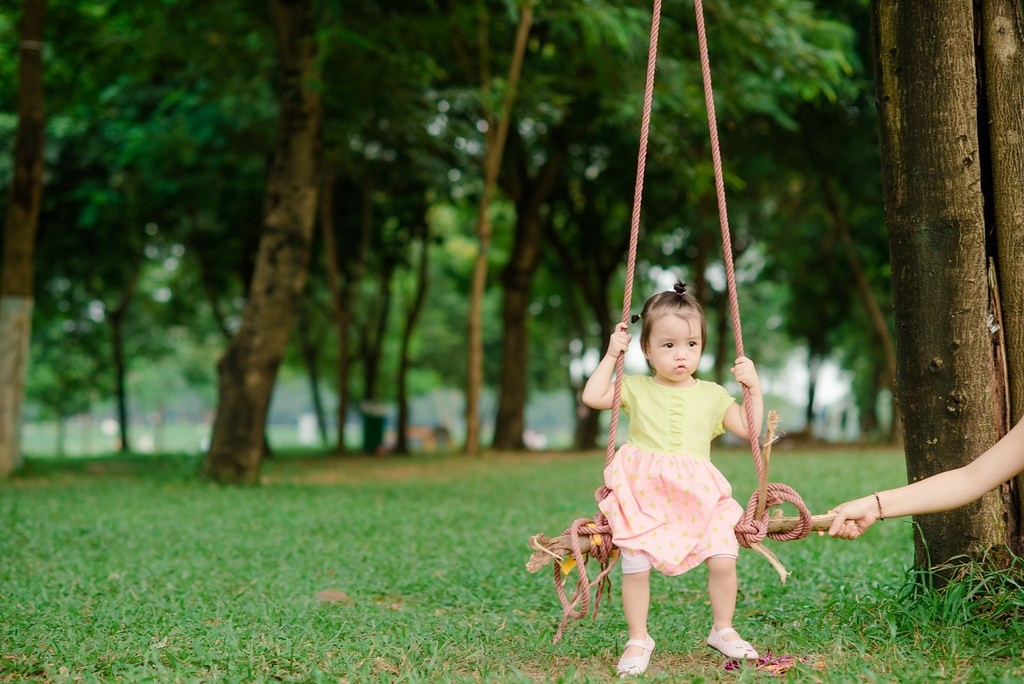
[873,492,884,521]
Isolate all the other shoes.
[706,624,760,660]
[616,632,656,678]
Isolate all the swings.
[525,0,859,578]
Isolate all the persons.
[817,415,1024,541]
[582,282,763,678]
